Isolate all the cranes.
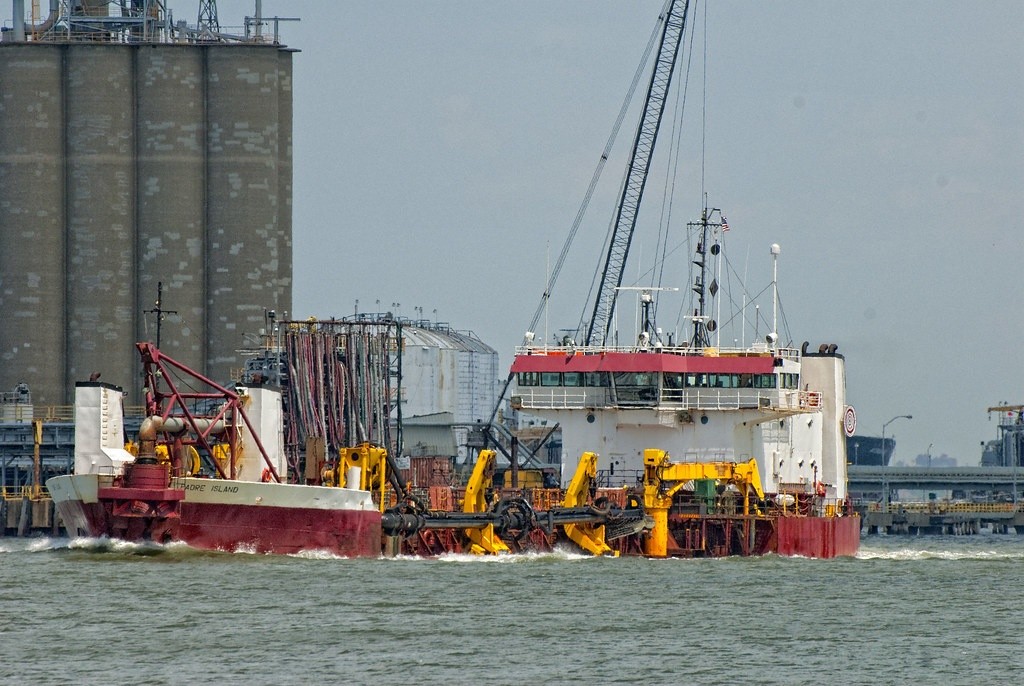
[986,404,1023,438]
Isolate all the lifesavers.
[817,482,827,496]
[806,393,818,406]
[262,469,271,483]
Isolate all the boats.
[44,0,864,564]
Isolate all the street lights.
[882,414,913,513]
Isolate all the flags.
[721,216,731,232]
